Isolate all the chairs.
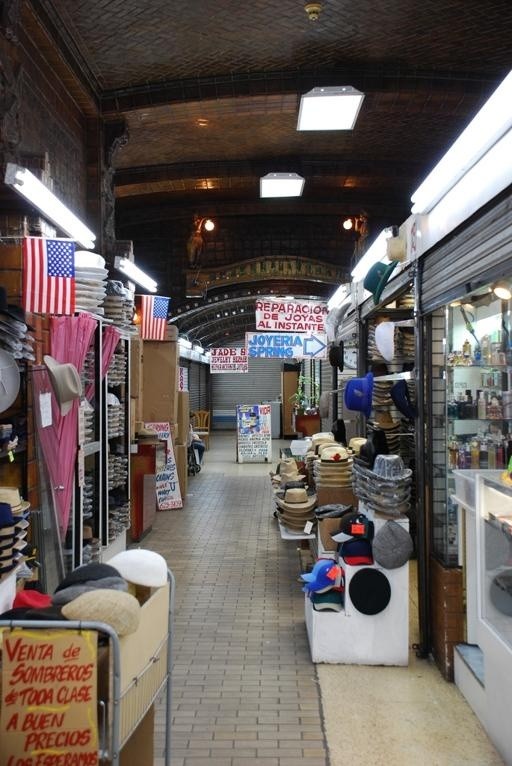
[192,410,211,451]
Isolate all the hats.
[272,411,413,529]
[329,260,414,411]
[372,519,414,570]
[329,511,372,566]
[350,568,391,615]
[83,526,98,547]
[1,244,136,547]
[298,558,345,612]
[0,548,168,637]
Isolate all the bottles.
[445,328,512,471]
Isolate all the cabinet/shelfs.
[62,315,133,571]
[304,504,411,667]
[430,293,512,570]
[356,307,415,439]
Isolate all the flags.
[20,236,77,317]
[140,293,171,342]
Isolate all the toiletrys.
[445,333,511,472]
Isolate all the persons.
[189,411,206,464]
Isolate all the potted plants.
[288,376,306,416]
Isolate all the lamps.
[258,172,306,199]
[5,162,98,252]
[494,275,512,300]
[113,255,159,293]
[294,83,364,132]
[196,215,215,232]
